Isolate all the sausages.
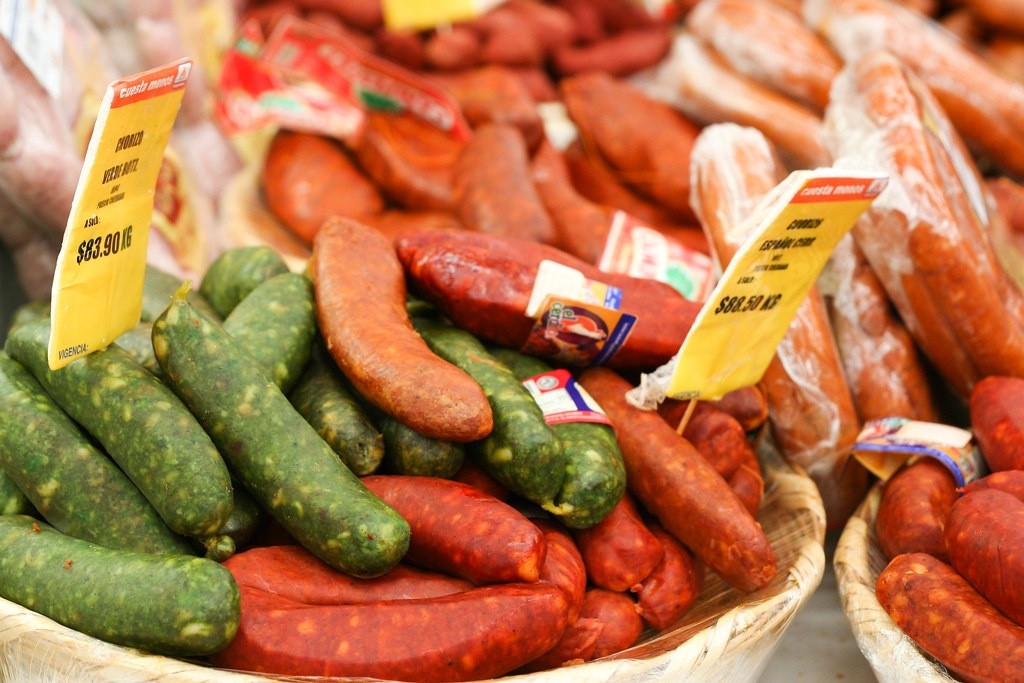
[0,0,1024,683]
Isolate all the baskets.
[832,477,958,683]
[0,462,826,683]
